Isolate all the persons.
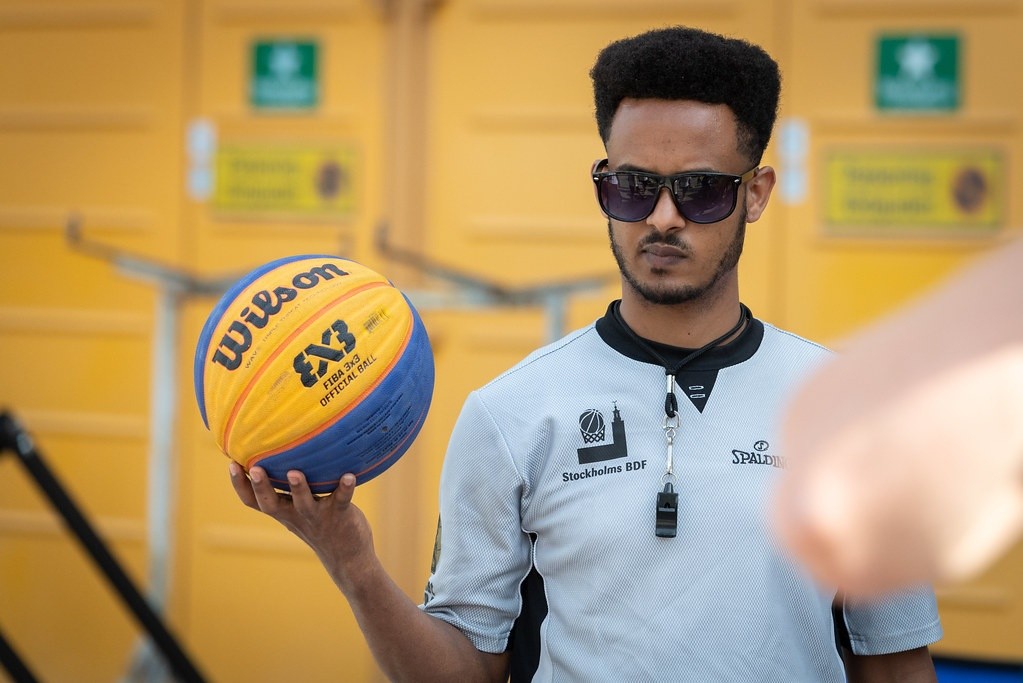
[227,26,943,683]
[679,176,692,199]
[627,174,649,200]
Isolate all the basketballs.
[192,253,440,500]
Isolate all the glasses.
[591,159,760,224]
